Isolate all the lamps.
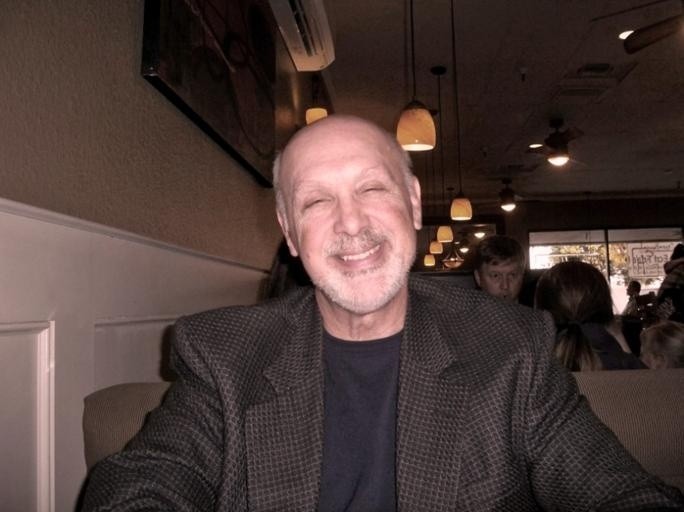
[396,0,474,270]
[497,176,518,212]
[543,119,570,168]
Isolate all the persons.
[474,235,684,372]
[76,115,684,512]
[257,235,313,302]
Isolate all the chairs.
[81,366,683,509]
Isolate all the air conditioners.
[267,0,336,73]
